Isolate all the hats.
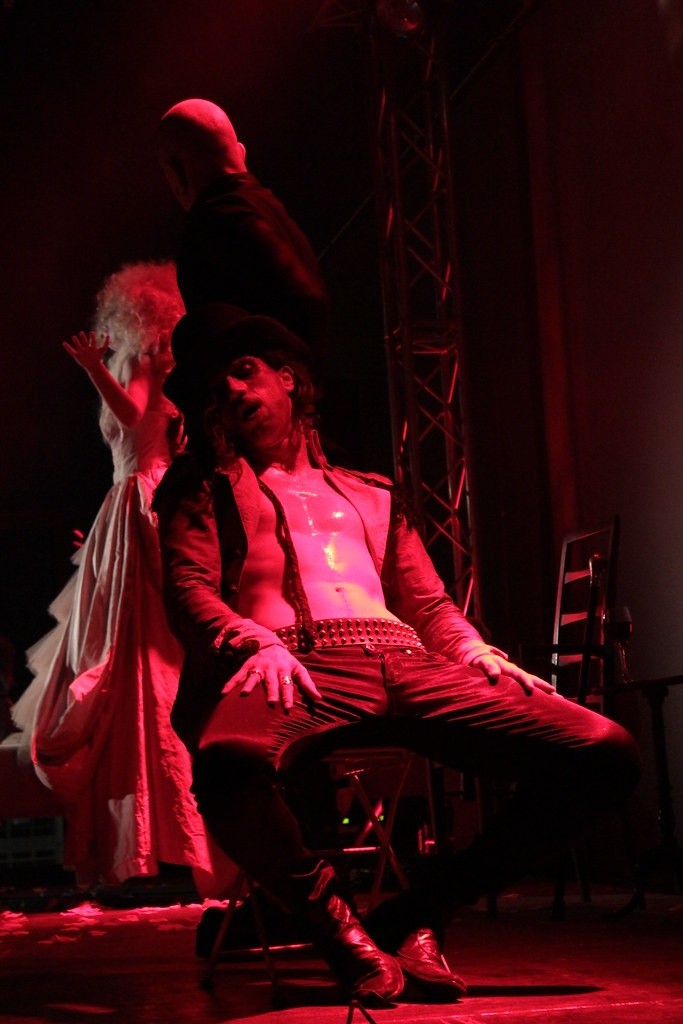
[163,296,315,427]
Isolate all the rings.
[280,676,295,687]
[249,669,263,681]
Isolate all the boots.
[301,894,403,1006]
[394,925,472,997]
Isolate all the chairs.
[196,743,424,1010]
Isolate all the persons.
[153,320,645,1005]
[0,97,331,905]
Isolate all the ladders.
[553,513,648,917]
